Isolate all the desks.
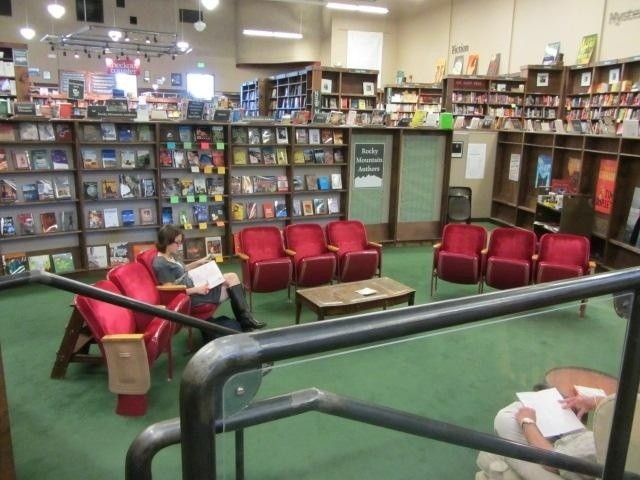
[294,275,418,326]
[543,365,620,414]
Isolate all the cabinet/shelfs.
[395,127,453,245]
[228,121,292,264]
[0,119,86,290]
[292,122,350,223]
[489,130,640,272]
[348,126,401,246]
[75,118,162,278]
[155,119,230,268]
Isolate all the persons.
[493,385,606,480]
[151,224,267,332]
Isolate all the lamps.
[18,0,222,64]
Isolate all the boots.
[227,284,267,332]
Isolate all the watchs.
[519,417,536,429]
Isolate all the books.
[515,387,585,438]
[187,260,226,290]
[345,34,640,135]
[0,57,231,277]
[231,79,345,220]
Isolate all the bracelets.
[594,396,597,406]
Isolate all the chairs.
[325,219,383,283]
[538,232,598,320]
[105,262,193,383]
[430,223,488,296]
[233,225,292,314]
[488,226,539,291]
[48,280,173,419]
[283,221,339,289]
[136,248,219,353]
